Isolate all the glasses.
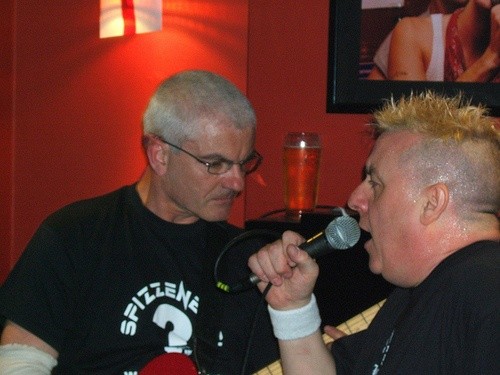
[156,138,263,176]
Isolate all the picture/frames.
[325,0,500,116]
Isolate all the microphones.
[231,215,361,290]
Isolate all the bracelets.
[268,293,322,340]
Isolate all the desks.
[245,208,372,277]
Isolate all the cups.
[284,131,320,218]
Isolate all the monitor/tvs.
[327,0,500,118]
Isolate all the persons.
[371,0,500,80]
[248,90,500,375]
[0,70,349,375]
